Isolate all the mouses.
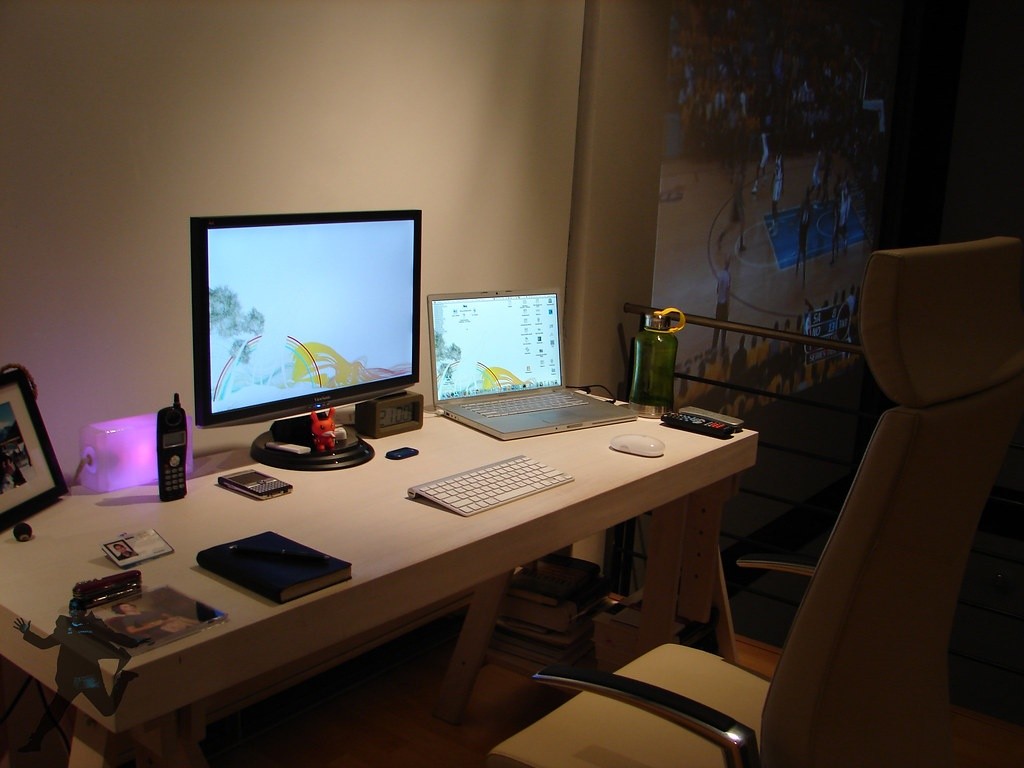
[610,433,667,458]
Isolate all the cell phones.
[218,470,294,499]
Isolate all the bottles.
[629,308,685,419]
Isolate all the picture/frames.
[0,368,70,534]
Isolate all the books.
[484,552,623,681]
[196,531,352,604]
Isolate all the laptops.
[426,288,637,441]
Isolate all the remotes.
[660,412,734,438]
[679,406,744,432]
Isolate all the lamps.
[72,413,194,496]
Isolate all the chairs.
[478,235,1024,768]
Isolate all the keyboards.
[408,453,575,517]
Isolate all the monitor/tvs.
[189,209,421,471]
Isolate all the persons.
[114,544,132,560]
[657,0,880,421]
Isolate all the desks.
[0,389,762,768]
